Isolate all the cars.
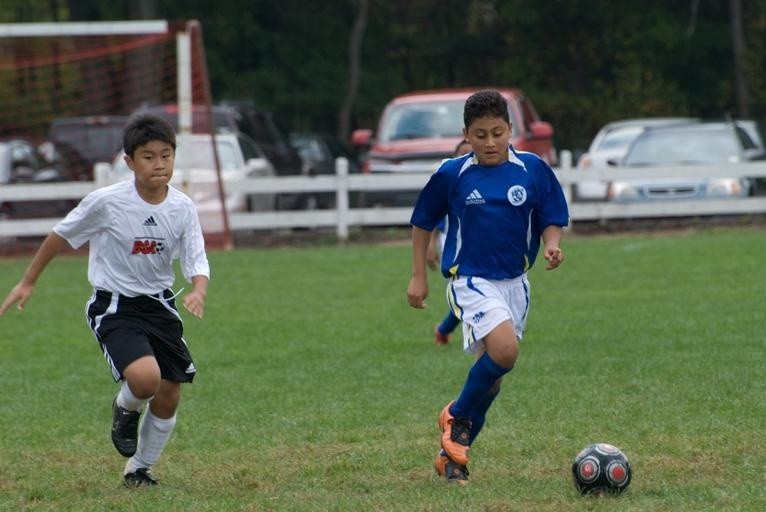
[570,111,766,231]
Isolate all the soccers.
[573,444,630,498]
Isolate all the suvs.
[348,84,562,241]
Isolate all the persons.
[408,88,571,486]
[0,111,212,489]
[423,135,476,345]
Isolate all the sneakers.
[111,393,143,456]
[438,398,469,463]
[435,325,451,345]
[121,454,157,489]
[433,450,469,486]
